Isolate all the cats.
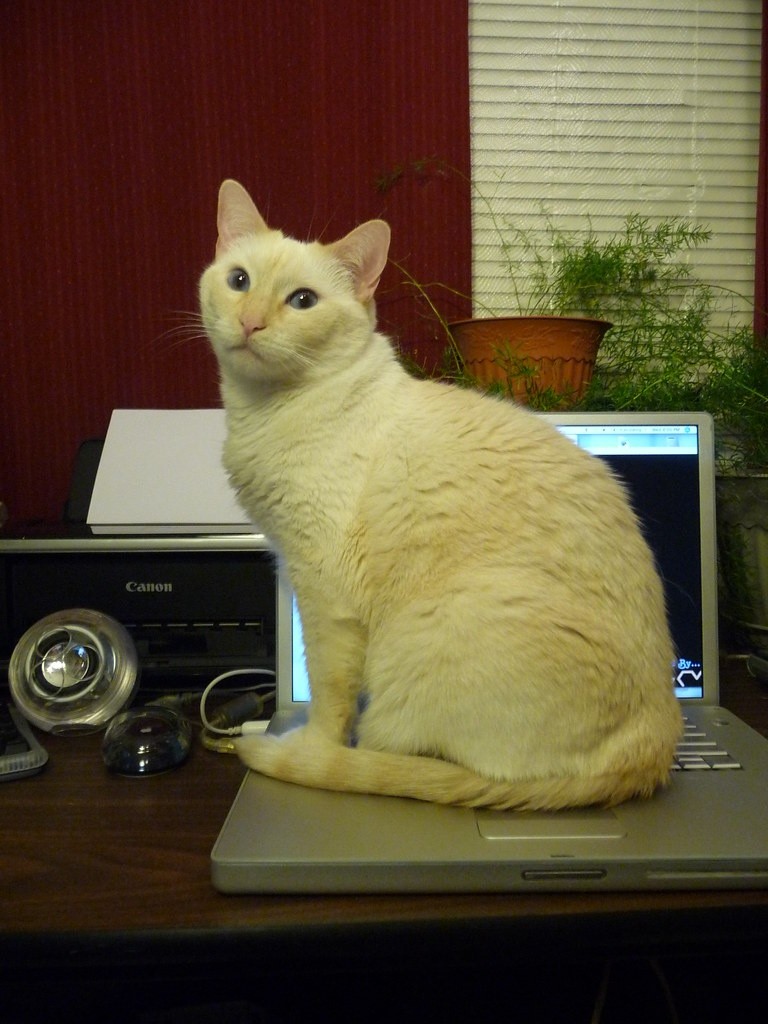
[199,180,683,809]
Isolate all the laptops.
[211,411,768,894]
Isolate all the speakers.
[8,607,140,738]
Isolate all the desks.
[0,664,768,970]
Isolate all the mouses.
[101,704,192,778]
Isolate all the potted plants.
[376,159,768,681]
